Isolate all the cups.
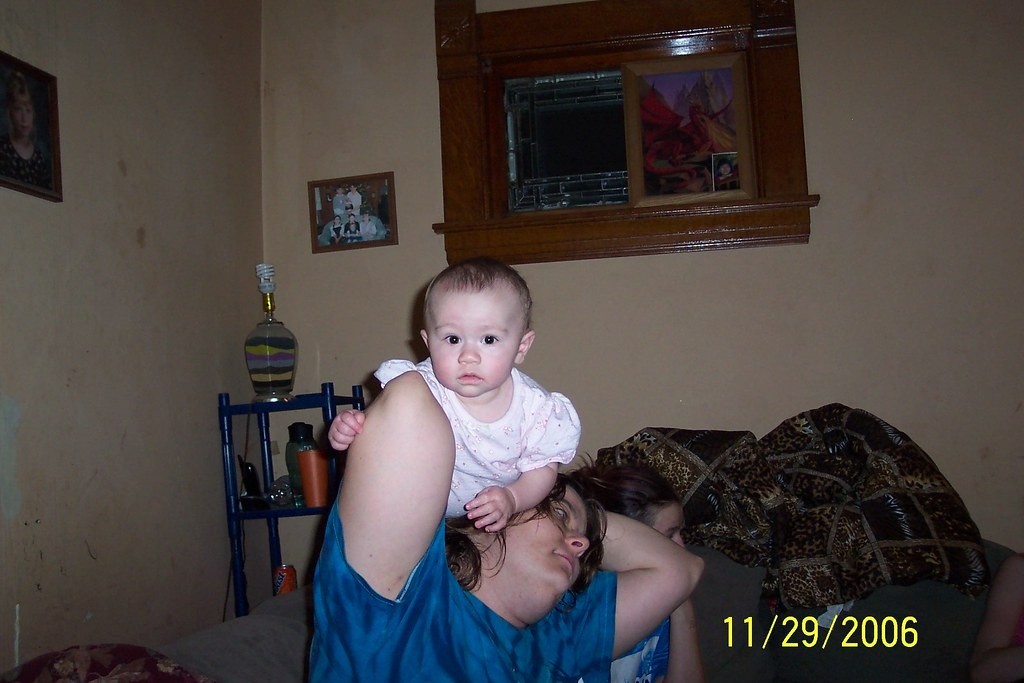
[294,449,328,507]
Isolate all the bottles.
[285,422,317,508]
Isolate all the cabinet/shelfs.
[217,381,364,617]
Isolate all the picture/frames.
[0,49,64,203]
[307,171,399,254]
[620,54,758,207]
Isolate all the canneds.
[273,564,297,596]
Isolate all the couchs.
[158,550,987,683]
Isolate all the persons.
[571,461,703,683]
[325,256,580,532]
[0,70,47,187]
[716,158,737,190]
[308,370,705,683]
[329,185,376,245]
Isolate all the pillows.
[770,577,985,683]
